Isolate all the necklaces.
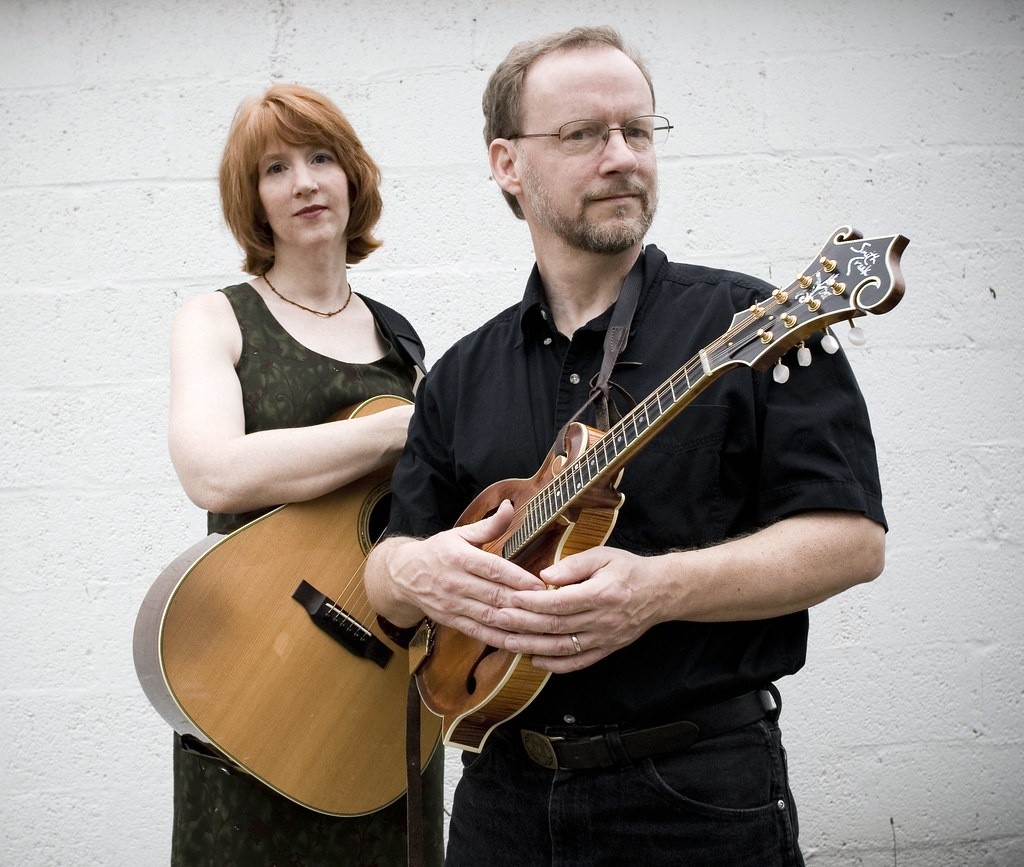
[263,272,352,317]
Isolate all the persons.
[361,27,887,867]
[168,81,446,867]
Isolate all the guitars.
[412,223,915,756]
[133,388,443,820]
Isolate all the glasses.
[508,114,674,157]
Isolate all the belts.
[481,683,777,771]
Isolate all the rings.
[570,634,584,653]
